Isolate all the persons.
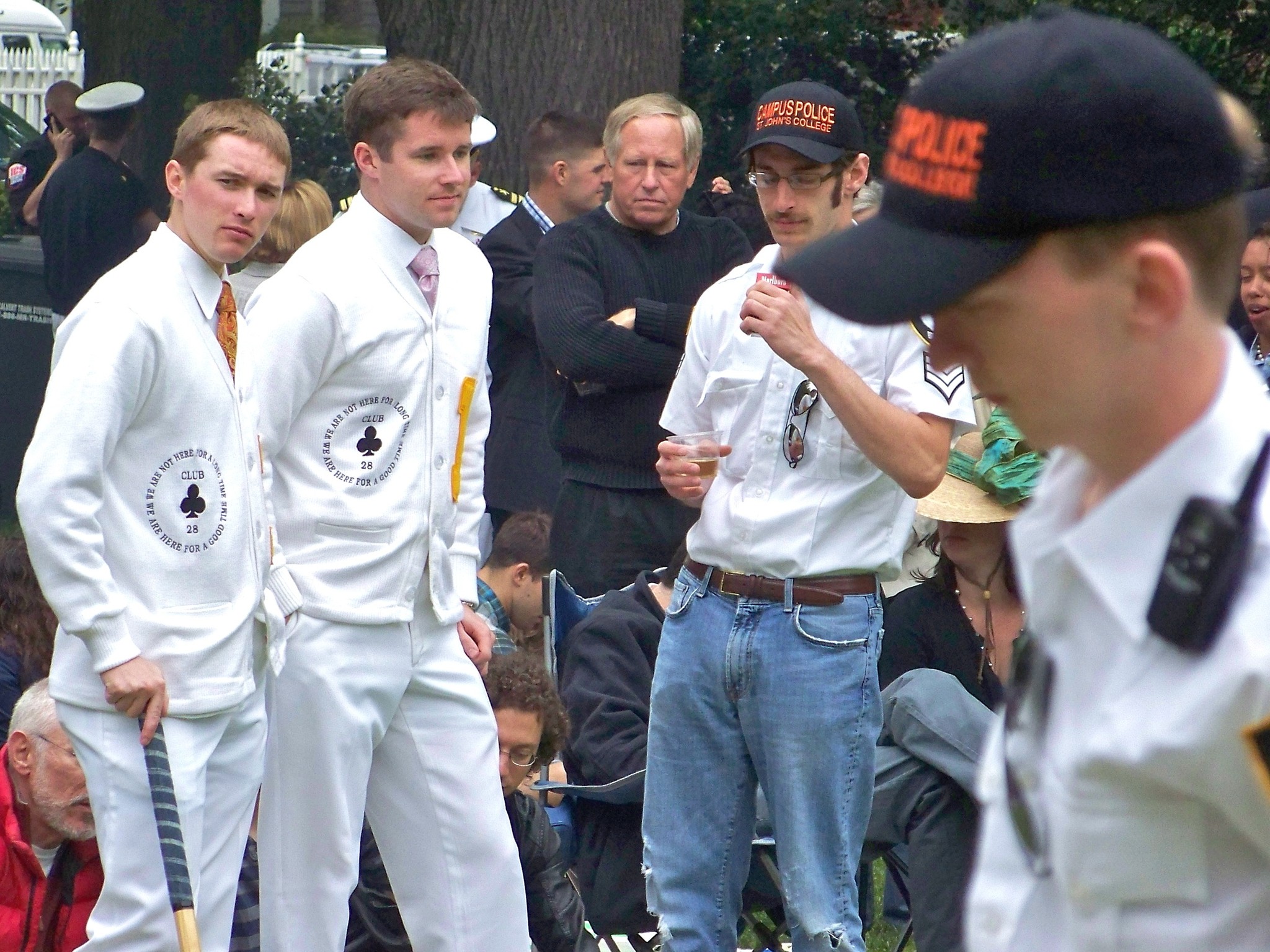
[5,80,87,229]
[642,76,976,952]
[344,652,600,952]
[556,539,999,952]
[693,101,777,252]
[877,431,1045,715]
[1237,221,1270,386]
[229,179,333,314]
[242,57,529,952]
[0,536,58,750]
[532,93,755,597]
[477,512,552,654]
[477,109,611,538]
[449,114,524,248]
[0,677,104,952]
[37,81,163,340]
[18,98,293,952]
[850,180,884,225]
[772,4,1270,952]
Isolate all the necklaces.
[1256,345,1264,361]
[955,590,1025,668]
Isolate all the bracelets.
[461,600,474,609]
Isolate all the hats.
[733,78,864,161]
[75,82,145,114]
[771,3,1269,326]
[468,115,497,153]
[915,404,1044,526]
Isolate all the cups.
[666,431,722,479]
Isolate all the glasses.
[35,733,82,769]
[497,743,539,767]
[783,379,818,468]
[744,156,837,191]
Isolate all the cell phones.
[43,113,64,133]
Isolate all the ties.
[216,281,237,385]
[410,246,439,316]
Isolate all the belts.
[684,555,877,607]
